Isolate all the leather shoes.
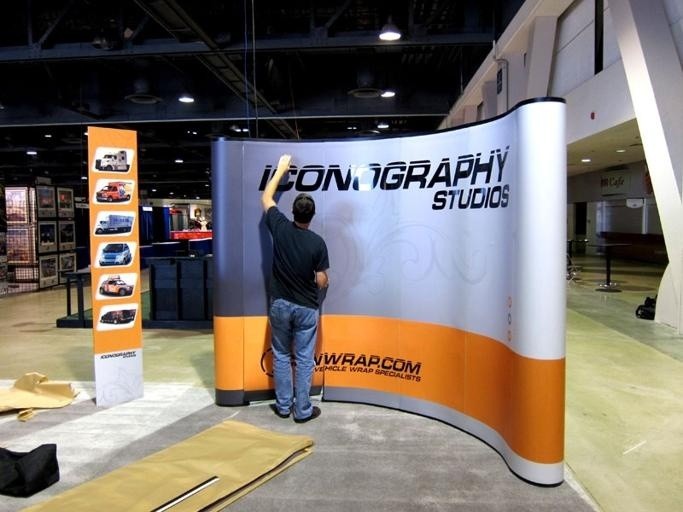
[295,406,322,423]
[279,403,295,418]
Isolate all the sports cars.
[99,309,136,324]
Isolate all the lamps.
[376,11,404,42]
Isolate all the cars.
[100,243,134,267]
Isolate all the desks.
[566,237,630,293]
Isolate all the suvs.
[96,276,132,298]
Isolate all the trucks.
[92,148,133,176]
[94,180,134,205]
[95,213,134,237]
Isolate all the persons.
[261,154,329,422]
